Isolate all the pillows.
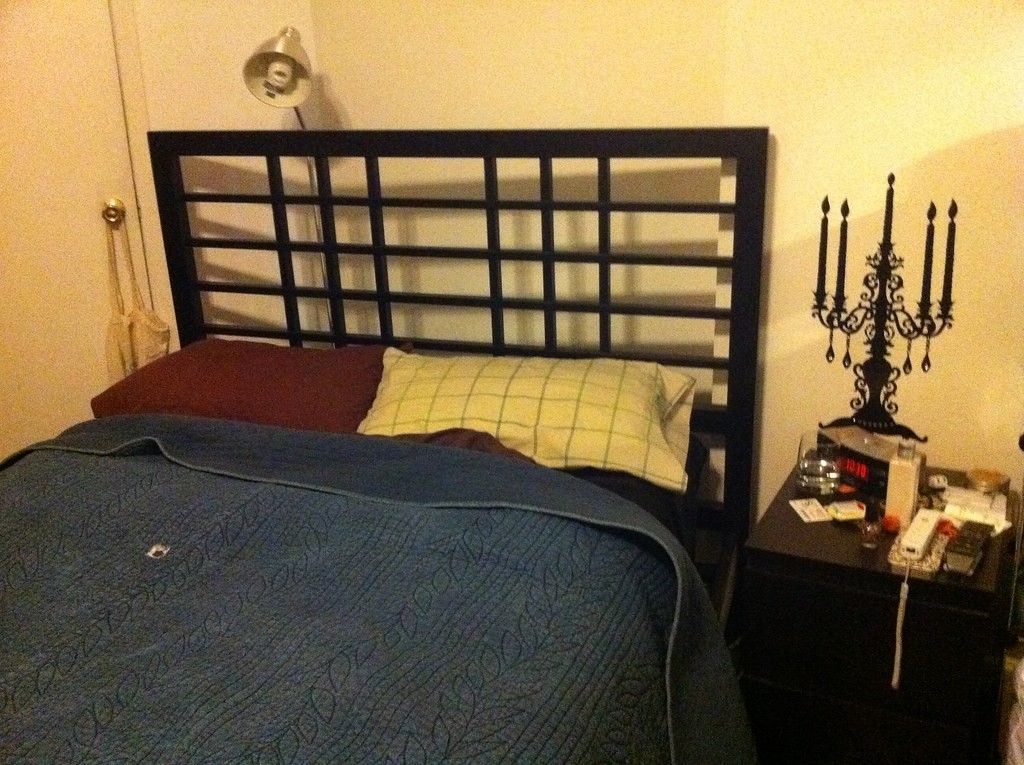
[354,346,699,495]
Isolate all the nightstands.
[736,451,1024,765]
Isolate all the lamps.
[244,26,340,348]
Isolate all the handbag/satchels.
[105,215,170,390]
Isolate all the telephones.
[901,508,939,560]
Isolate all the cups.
[796,430,841,495]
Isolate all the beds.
[0,128,768,765]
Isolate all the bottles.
[859,502,881,549]
